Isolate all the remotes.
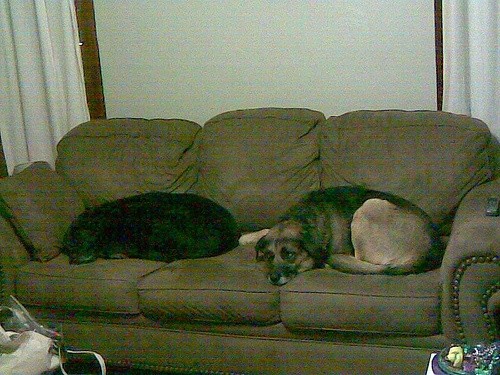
[485,197,500,216]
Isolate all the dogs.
[58,191,241,266]
[254,186,443,287]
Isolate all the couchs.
[0,108,500,375]
